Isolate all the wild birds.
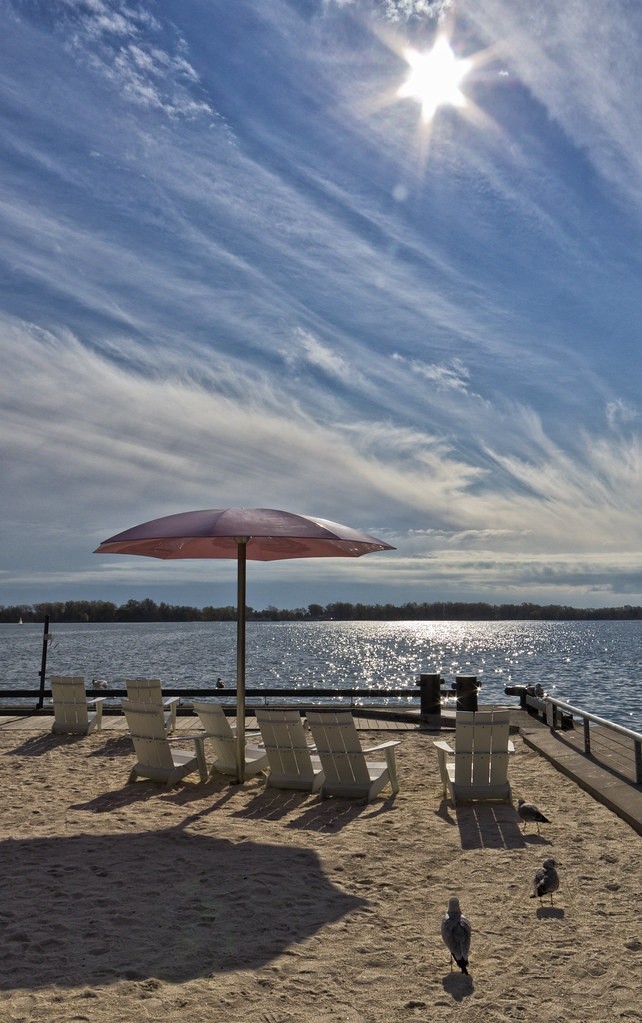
[517,798,552,833]
[92,679,108,690]
[441,897,472,976]
[216,678,224,688]
[529,859,563,905]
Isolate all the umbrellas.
[93,508,397,781]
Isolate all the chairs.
[306,711,401,803]
[48,675,106,736]
[254,710,325,793]
[434,713,515,808]
[192,702,269,784]
[122,679,180,738]
[121,699,210,791]
